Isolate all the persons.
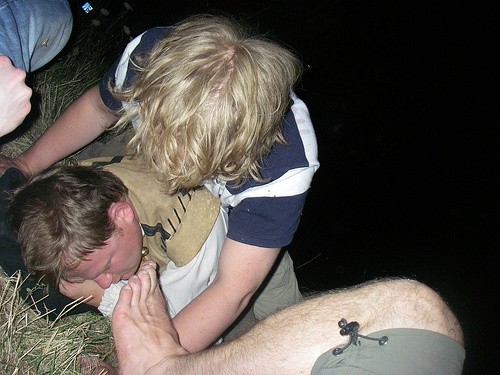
[1,0,95,148]
[0,16,326,357]
[105,258,467,375]
[0,156,301,333]
